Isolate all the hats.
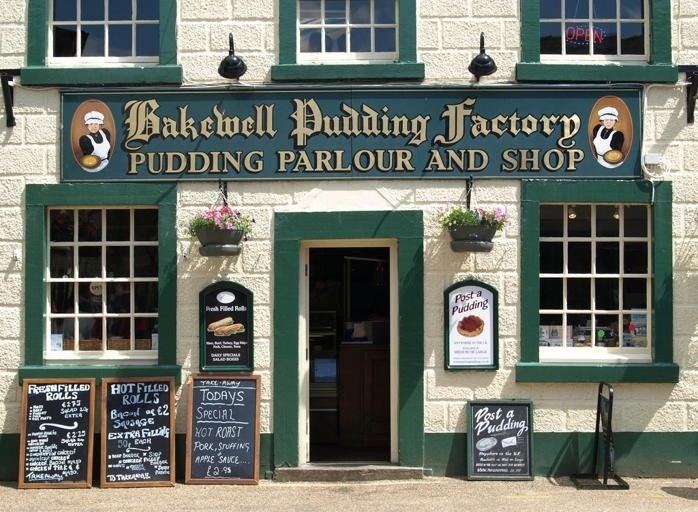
[598,107,618,121]
[84,111,104,125]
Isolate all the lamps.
[468,32,497,82]
[218,33,247,81]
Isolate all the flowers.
[437,209,507,238]
[189,207,256,241]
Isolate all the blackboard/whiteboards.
[19,374,261,489]
[467,399,534,481]
[592,381,615,484]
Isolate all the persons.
[63,290,98,339]
[78,110,111,173]
[592,106,624,168]
[111,282,148,339]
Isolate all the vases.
[195,229,243,255]
[448,225,496,252]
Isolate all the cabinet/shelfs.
[339,344,390,448]
[309,311,339,444]
